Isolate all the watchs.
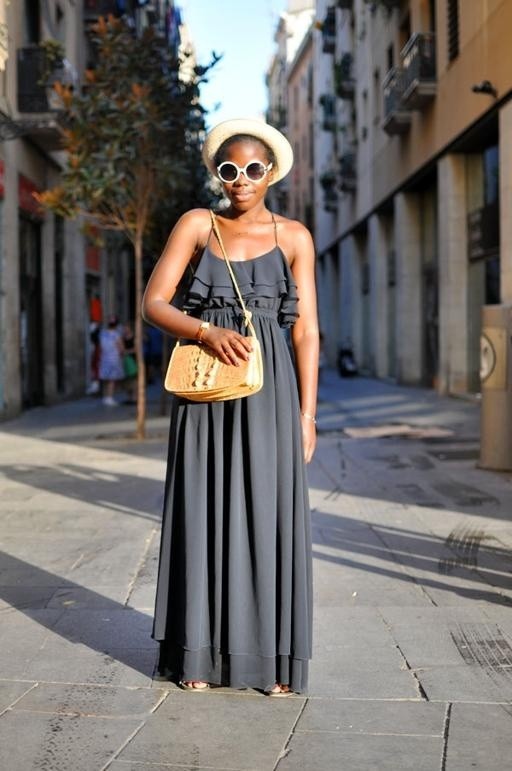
[197,321,211,344]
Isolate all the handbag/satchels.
[124,354,137,376]
[165,333,264,402]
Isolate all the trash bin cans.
[476,304,512,471]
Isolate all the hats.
[202,119,294,189]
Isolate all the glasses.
[212,160,272,183]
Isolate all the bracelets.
[302,413,317,423]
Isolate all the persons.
[141,117,321,697]
[86,311,164,408]
